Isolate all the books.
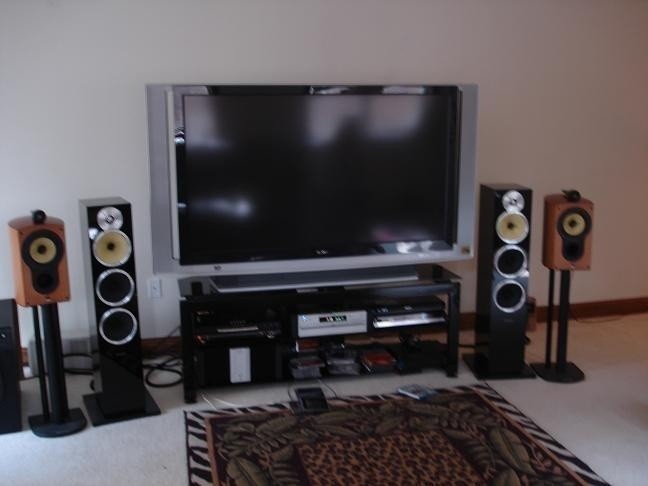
[288,350,437,416]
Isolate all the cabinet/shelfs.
[179,266,466,399]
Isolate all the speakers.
[462,181,536,380]
[542,193,594,272]
[79,196,162,427]
[7,215,71,308]
[0,298,22,434]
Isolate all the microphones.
[30,209,48,225]
[561,188,580,203]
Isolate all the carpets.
[179,378,616,485]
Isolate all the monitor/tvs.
[145,81,480,294]
